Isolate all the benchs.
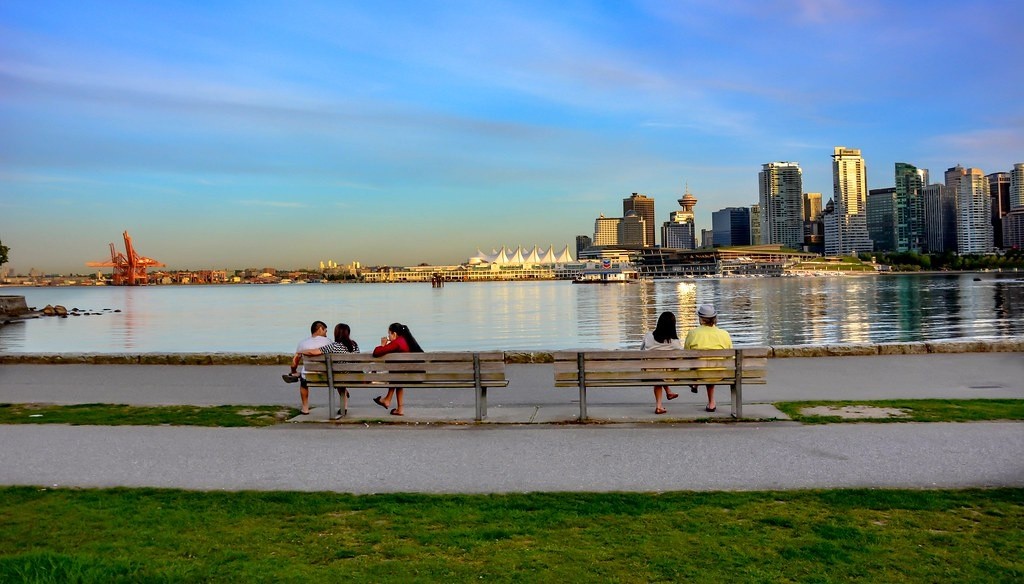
[303,350,513,418]
[552,348,767,422]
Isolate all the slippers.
[391,408,404,416]
[300,409,309,415]
[706,404,716,412]
[373,396,388,409]
[282,375,298,383]
[691,385,698,393]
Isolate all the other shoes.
[338,408,347,416]
[666,393,679,400]
[655,408,667,414]
[347,392,350,398]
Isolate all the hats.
[697,304,717,317]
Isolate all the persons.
[684,304,734,412]
[282,321,334,414]
[296,324,360,415]
[639,311,682,413]
[372,323,424,416]
